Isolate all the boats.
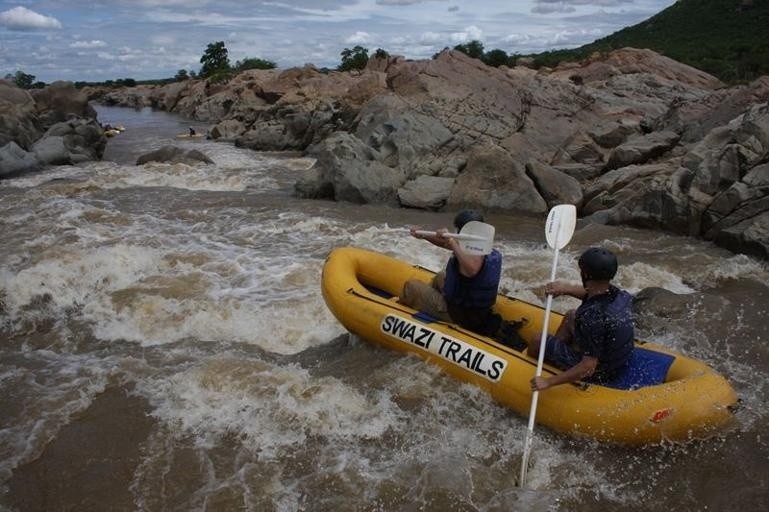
[321,244,741,450]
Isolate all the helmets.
[578,248,618,281]
[454,210,483,231]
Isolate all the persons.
[189,128,196,138]
[526,247,635,392]
[397,209,502,329]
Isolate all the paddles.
[414,221,495,254]
[521,205,577,486]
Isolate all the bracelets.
[446,237,454,243]
